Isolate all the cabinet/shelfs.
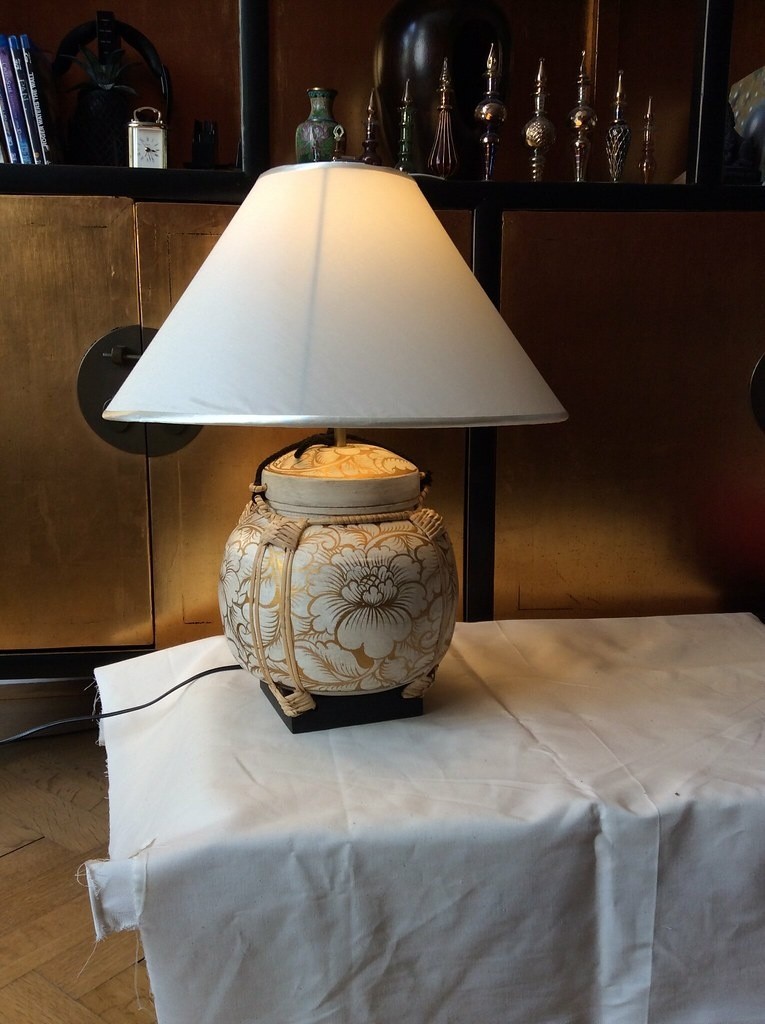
[1,0,765,683]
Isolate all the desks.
[78,612,765,1024]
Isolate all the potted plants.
[40,42,144,168]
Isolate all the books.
[0,32,56,165]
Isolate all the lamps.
[103,160,571,734]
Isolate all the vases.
[295,87,347,164]
[374,0,514,181]
